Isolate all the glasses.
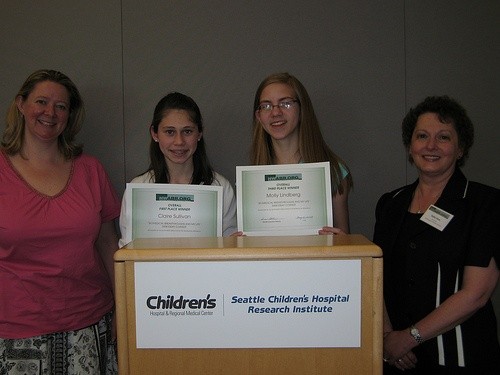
[257,98,300,111]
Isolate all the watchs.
[410,325,423,343]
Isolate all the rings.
[397,359,401,362]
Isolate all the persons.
[0,70,120,375]
[117,92,236,247]
[374,96,500,375]
[232,71,351,237]
